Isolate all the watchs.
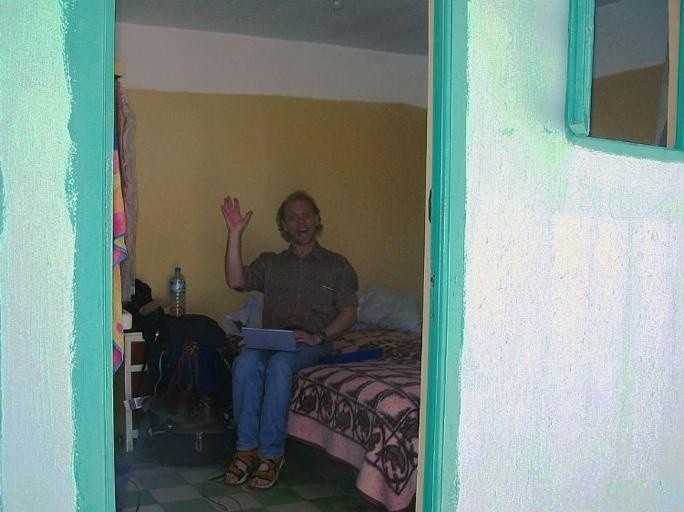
[317,327,327,343]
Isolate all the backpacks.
[134,312,238,469]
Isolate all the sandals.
[249,456,288,491]
[224,449,259,487]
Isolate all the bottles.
[168,266,186,316]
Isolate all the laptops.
[242,328,304,352]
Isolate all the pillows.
[223,291,264,331]
[357,282,420,334]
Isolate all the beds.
[224,327,420,512]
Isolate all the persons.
[218,188,359,490]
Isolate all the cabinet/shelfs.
[112,328,158,452]
